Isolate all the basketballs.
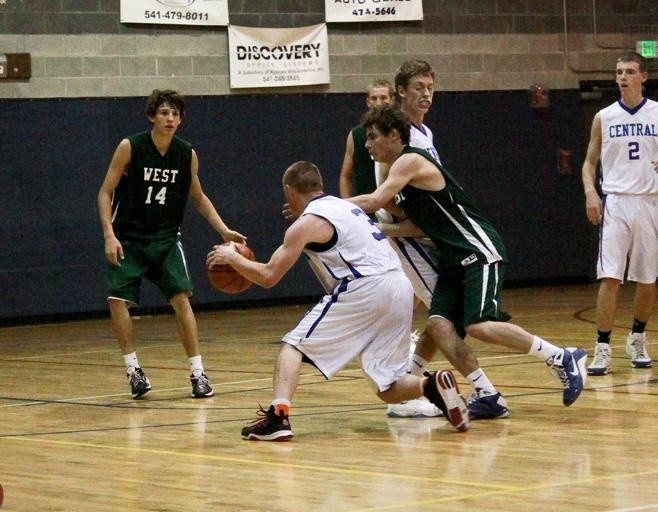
[206,243,256,294]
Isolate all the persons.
[374,58,444,421]
[206,161,470,443]
[339,79,396,201]
[581,52,658,377]
[96,88,247,402]
[282,106,588,421]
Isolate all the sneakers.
[190,369,215,399]
[586,341,614,376]
[126,366,152,400]
[422,369,470,432]
[546,346,588,407]
[464,389,511,421]
[625,330,653,368]
[386,394,443,418]
[240,402,294,442]
